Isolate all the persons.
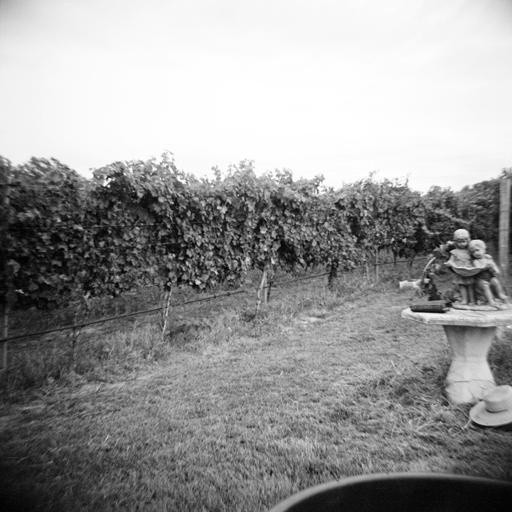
[444,229,508,307]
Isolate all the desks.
[406,306,512,408]
